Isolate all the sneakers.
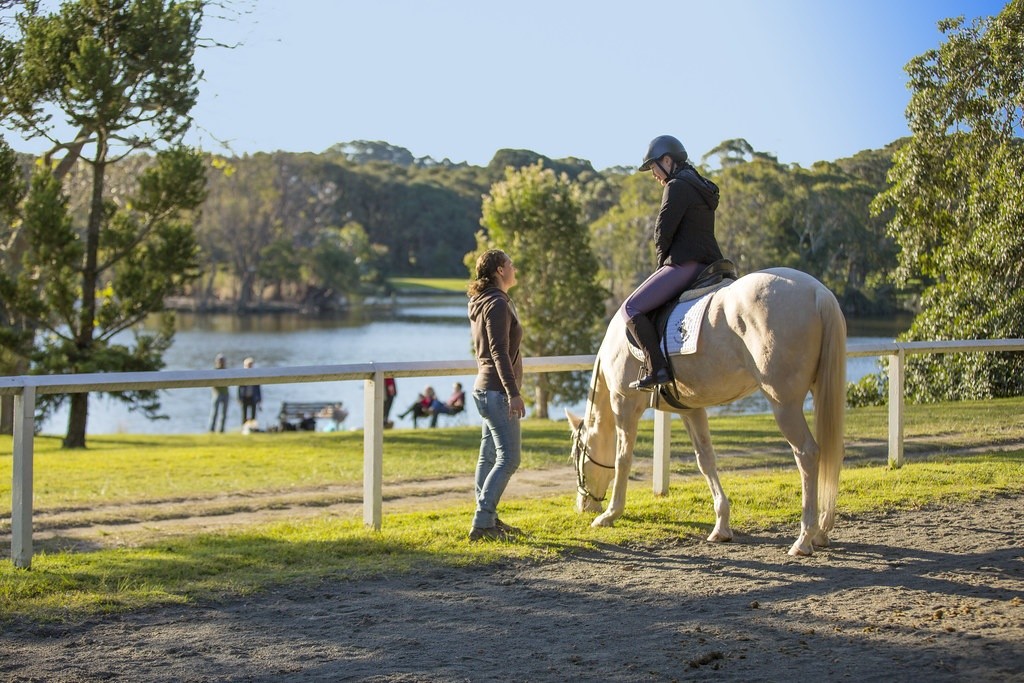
[469,527,515,542]
[495,517,521,535]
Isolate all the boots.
[626,313,672,389]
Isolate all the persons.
[420,382,464,428]
[209,353,229,432]
[621,134,723,390]
[396,385,434,428]
[383,378,396,428]
[466,248,526,542]
[236,356,262,425]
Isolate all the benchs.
[277,400,344,432]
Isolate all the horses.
[563,266,848,557]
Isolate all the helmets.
[638,135,688,172]
